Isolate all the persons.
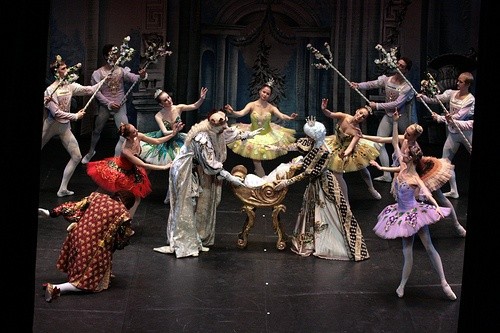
[224,76,298,179]
[37,45,475,303]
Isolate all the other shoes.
[42,283,60,302]
